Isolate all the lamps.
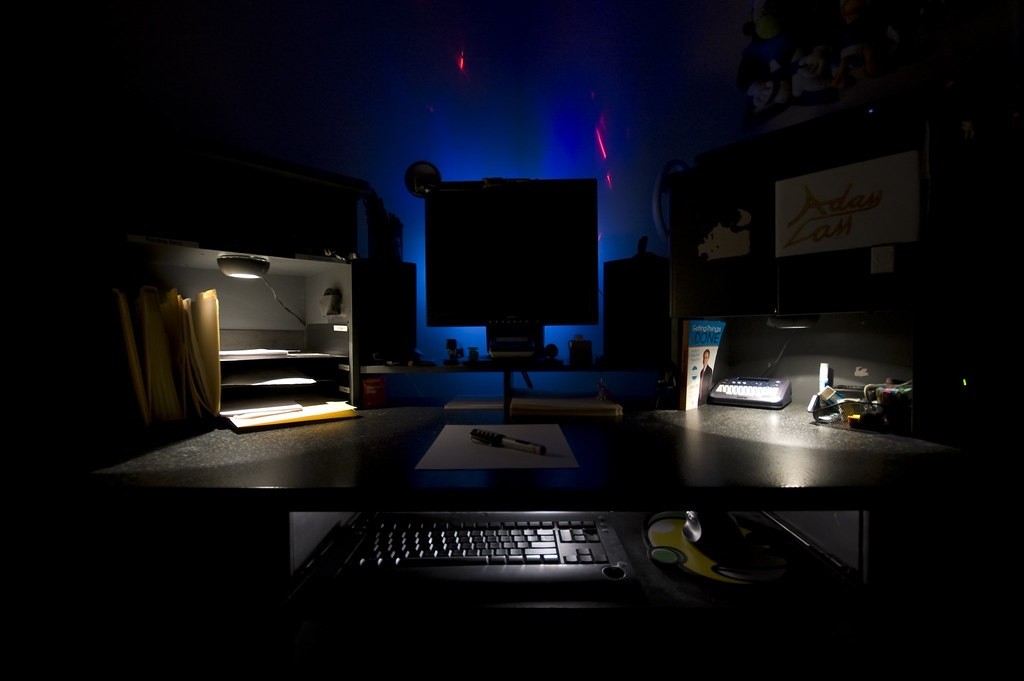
[766,314,821,330]
[216,257,270,280]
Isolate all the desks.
[80,407,964,681]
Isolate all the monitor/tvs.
[425,178,599,367]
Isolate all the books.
[114,287,220,427]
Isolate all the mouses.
[684,503,750,570]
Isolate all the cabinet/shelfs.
[669,85,934,437]
[96,133,370,445]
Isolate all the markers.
[469,427,547,456]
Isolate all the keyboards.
[336,513,628,590]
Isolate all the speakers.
[603,253,672,372]
[348,258,417,365]
